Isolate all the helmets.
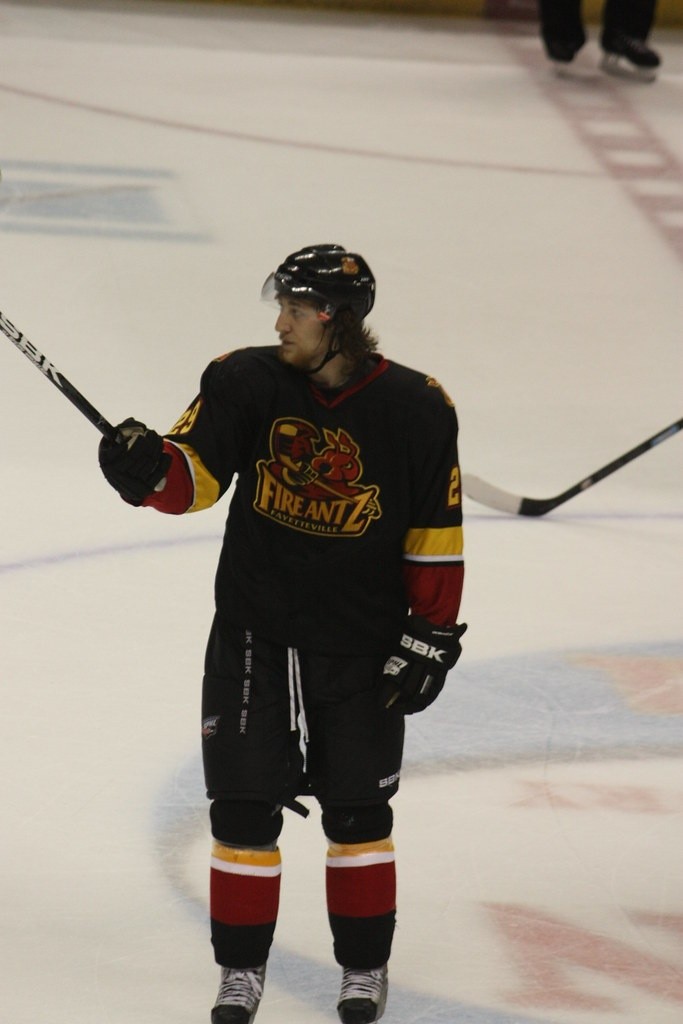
[261,244,375,316]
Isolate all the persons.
[97,244,469,1024]
[536,0,661,83]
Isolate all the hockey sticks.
[460,421,683,516]
[0,309,125,446]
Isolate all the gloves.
[99,418,170,504]
[381,621,468,717]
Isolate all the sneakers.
[210,969,266,1023]
[339,968,389,1024]
